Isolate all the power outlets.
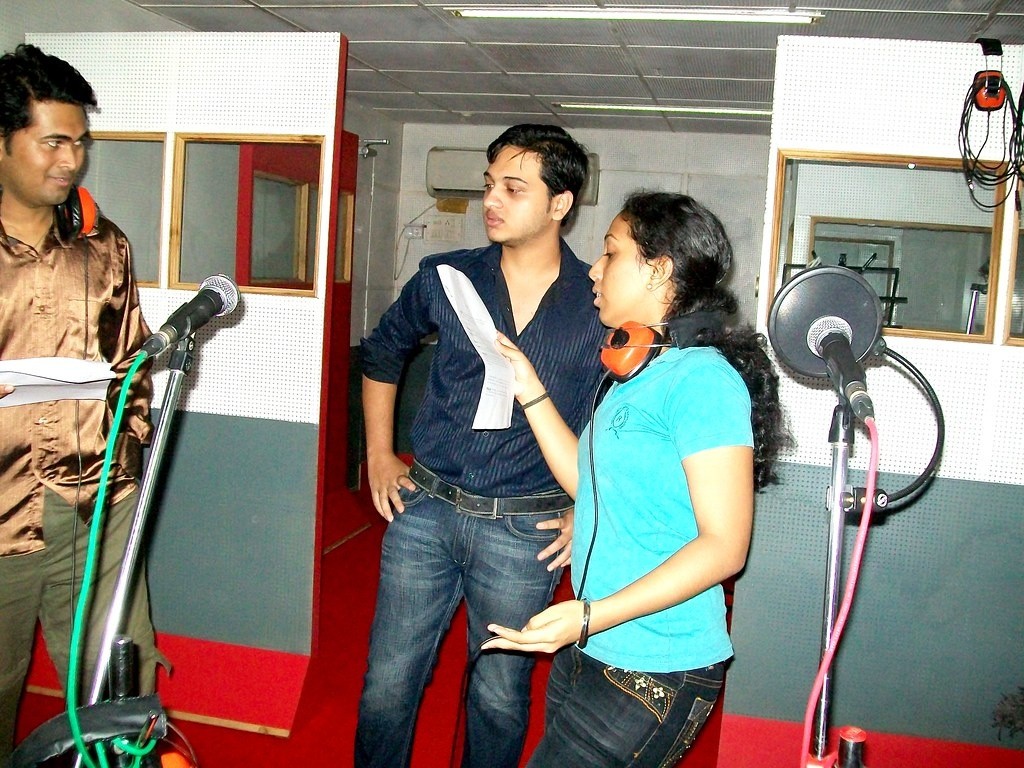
[405,226,424,239]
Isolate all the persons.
[479,190,789,767]
[358,121,615,768]
[0,43,170,767]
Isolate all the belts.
[407,459,575,520]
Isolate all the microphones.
[140,273,242,358]
[806,316,874,427]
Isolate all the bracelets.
[578,599,594,649]
[521,392,551,411]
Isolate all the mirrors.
[767,148,1008,344]
[1003,163,1024,347]
[809,215,992,335]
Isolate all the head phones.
[599,310,728,384]
[55,184,101,242]
[972,38,1007,111]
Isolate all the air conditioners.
[426,146,600,206]
